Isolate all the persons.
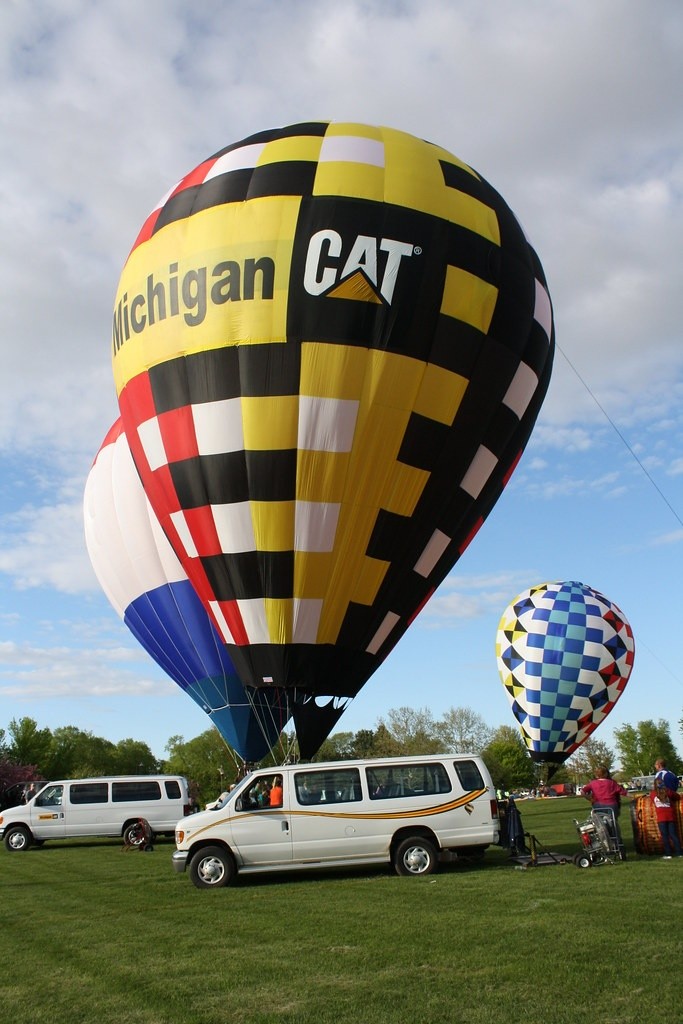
[624,780,641,789]
[654,757,679,792]
[249,783,270,807]
[270,780,283,806]
[650,778,683,860]
[25,782,37,803]
[580,765,636,846]
[296,776,323,801]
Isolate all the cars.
[0,781,60,811]
[618,782,637,790]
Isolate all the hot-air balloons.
[497,579,636,798]
[81,416,293,795]
[113,119,554,765]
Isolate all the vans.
[172,752,501,888]
[0,774,192,851]
[520,790,529,796]
[550,784,570,795]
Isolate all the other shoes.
[662,855,671,860]
